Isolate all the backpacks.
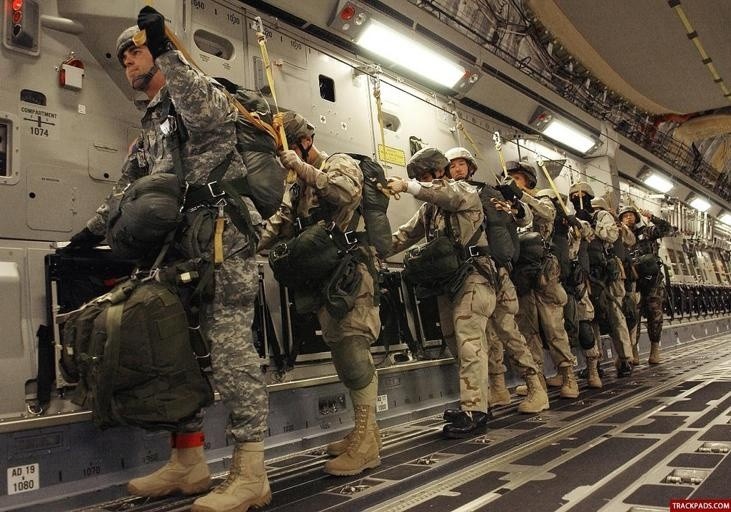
[316,151,394,260]
[161,74,285,221]
[441,174,519,271]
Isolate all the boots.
[322,375,381,475]
[547,342,663,399]
[516,372,551,414]
[488,373,512,409]
[191,439,272,512]
[128,440,211,496]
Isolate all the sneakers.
[441,408,487,440]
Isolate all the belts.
[458,242,491,260]
[182,177,252,211]
[332,229,366,250]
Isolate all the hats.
[501,159,644,225]
[407,144,450,182]
[444,146,480,183]
[279,111,315,142]
[115,23,142,67]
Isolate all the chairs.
[21,234,730,424]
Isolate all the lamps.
[716,207,731,227]
[634,165,675,195]
[684,191,714,214]
[528,105,604,157]
[325,0,484,105]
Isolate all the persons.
[536,188,603,391]
[257,107,382,476]
[447,147,550,414]
[386,147,497,439]
[616,205,671,365]
[566,182,634,378]
[71,7,272,507]
[500,158,580,399]
[586,197,636,371]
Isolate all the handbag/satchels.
[268,218,345,297]
[56,257,216,434]
[400,231,665,301]
[97,171,183,262]
